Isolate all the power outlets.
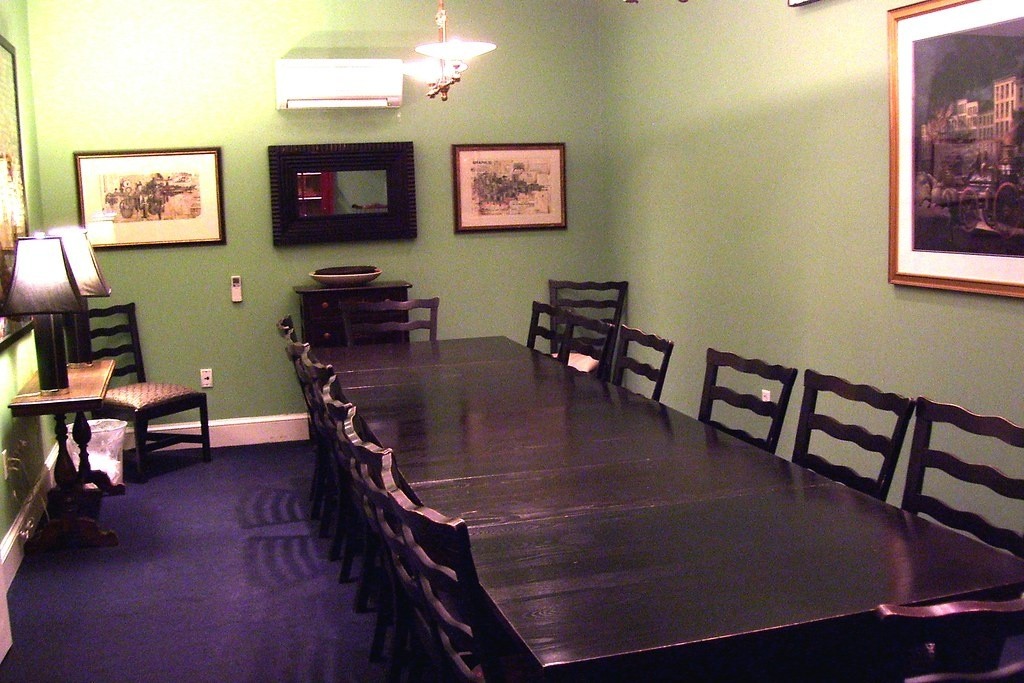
[2,449,11,480]
[200,369,214,388]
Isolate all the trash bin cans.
[66,418,128,492]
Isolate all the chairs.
[901,396,1024,560]
[380,453,540,683]
[343,406,409,683]
[699,348,797,455]
[75,302,212,482]
[560,309,617,381]
[276,314,326,520]
[526,300,572,361]
[549,279,628,376]
[301,345,347,563]
[322,374,379,613]
[611,323,674,402]
[338,297,439,347]
[792,368,916,502]
[873,594,1024,683]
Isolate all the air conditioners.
[274,59,403,110]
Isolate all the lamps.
[0,235,88,397]
[398,0,497,101]
[30,226,111,368]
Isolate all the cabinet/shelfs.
[292,280,413,345]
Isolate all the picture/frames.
[268,142,418,247]
[0,34,34,356]
[451,142,568,234]
[72,147,227,252]
[887,0,1024,299]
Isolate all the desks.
[309,335,1024,683]
[9,360,116,555]
